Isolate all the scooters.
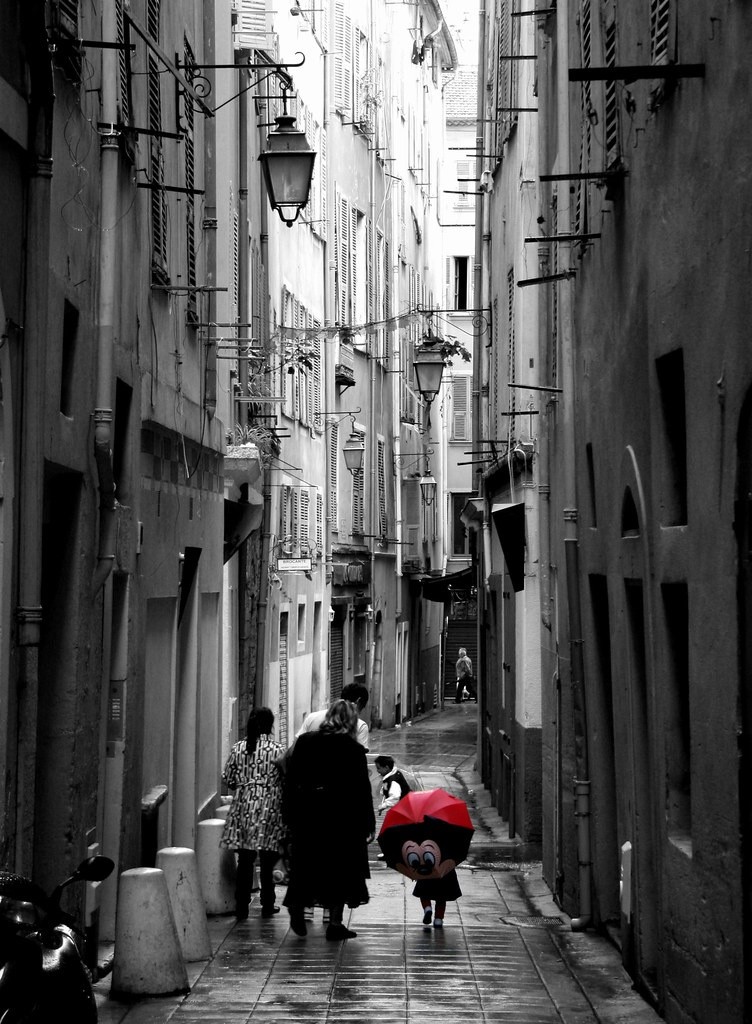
[0,854,115,1024]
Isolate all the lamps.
[392,448,437,505]
[174,52,317,228]
[413,301,492,406]
[314,408,364,477]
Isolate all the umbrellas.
[366,755,424,815]
[376,789,476,881]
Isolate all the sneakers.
[433,918,443,927]
[423,907,432,924]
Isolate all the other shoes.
[235,909,249,920]
[261,905,280,916]
[288,907,308,937]
[326,924,357,941]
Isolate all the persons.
[218,707,286,920]
[287,684,369,923]
[452,648,477,704]
[374,756,411,860]
[412,870,462,928]
[283,699,370,940]
